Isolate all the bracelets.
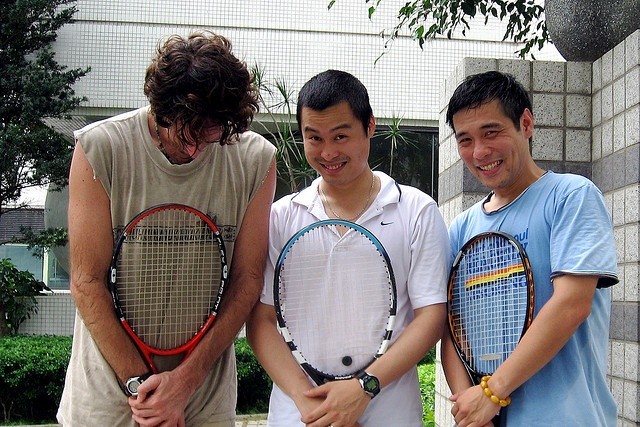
[478,374,512,408]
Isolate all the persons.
[437,69,621,426]
[54,23,278,426]
[243,68,453,426]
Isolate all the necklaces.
[146,110,192,166]
[318,172,379,221]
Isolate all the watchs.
[353,369,380,399]
[120,372,155,397]
